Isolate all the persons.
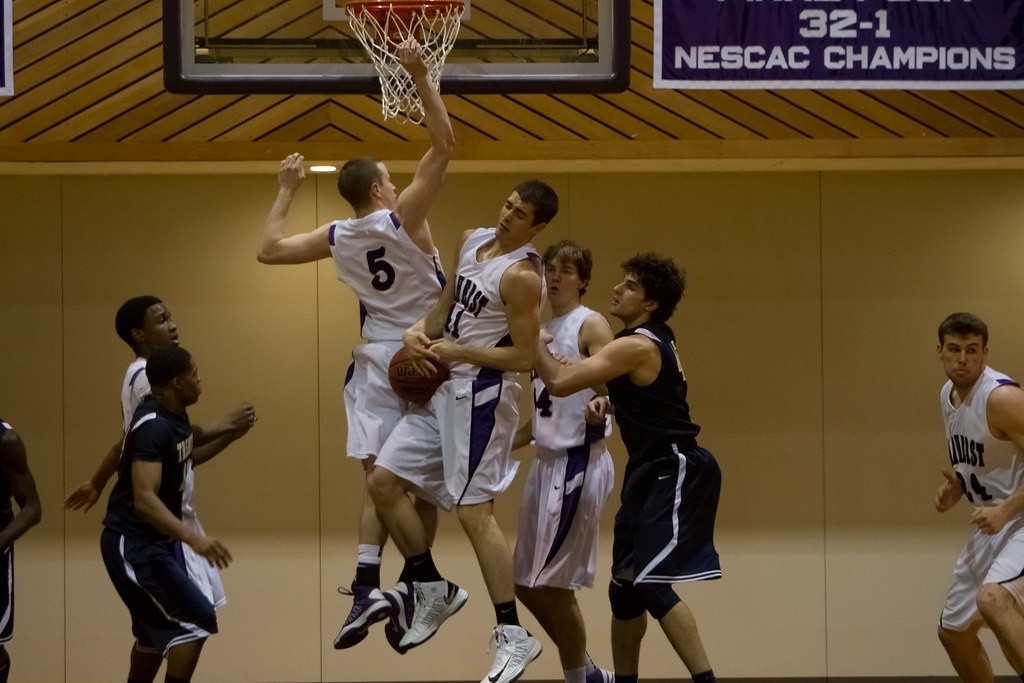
[935,312,1023,683]
[258,39,722,683]
[0,418,43,683]
[61,292,258,683]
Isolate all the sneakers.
[382,582,420,654]
[586,667,615,683]
[333,580,392,649]
[479,625,542,683]
[398,581,469,649]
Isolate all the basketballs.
[388,343,450,403]
